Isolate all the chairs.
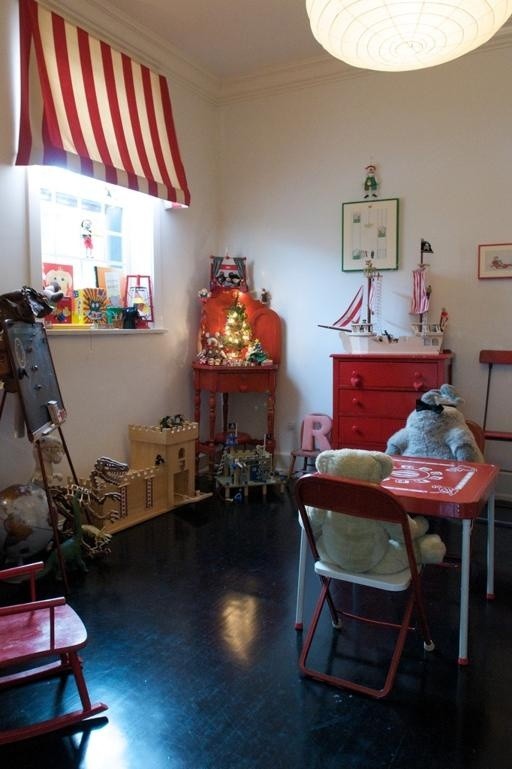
[291,476,436,699]
[464,421,485,455]
[479,349,512,524]
[0,561,108,744]
[287,414,333,480]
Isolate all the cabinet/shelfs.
[330,353,455,455]
[192,361,278,481]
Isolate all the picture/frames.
[478,243,511,279]
[342,198,399,271]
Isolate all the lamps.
[303,0,512,71]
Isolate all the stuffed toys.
[298,447,447,575]
[384,383,485,464]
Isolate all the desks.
[293,455,502,664]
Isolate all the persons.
[363,165,381,198]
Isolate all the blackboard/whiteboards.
[5,321,67,444]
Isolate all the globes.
[0,484,58,583]
